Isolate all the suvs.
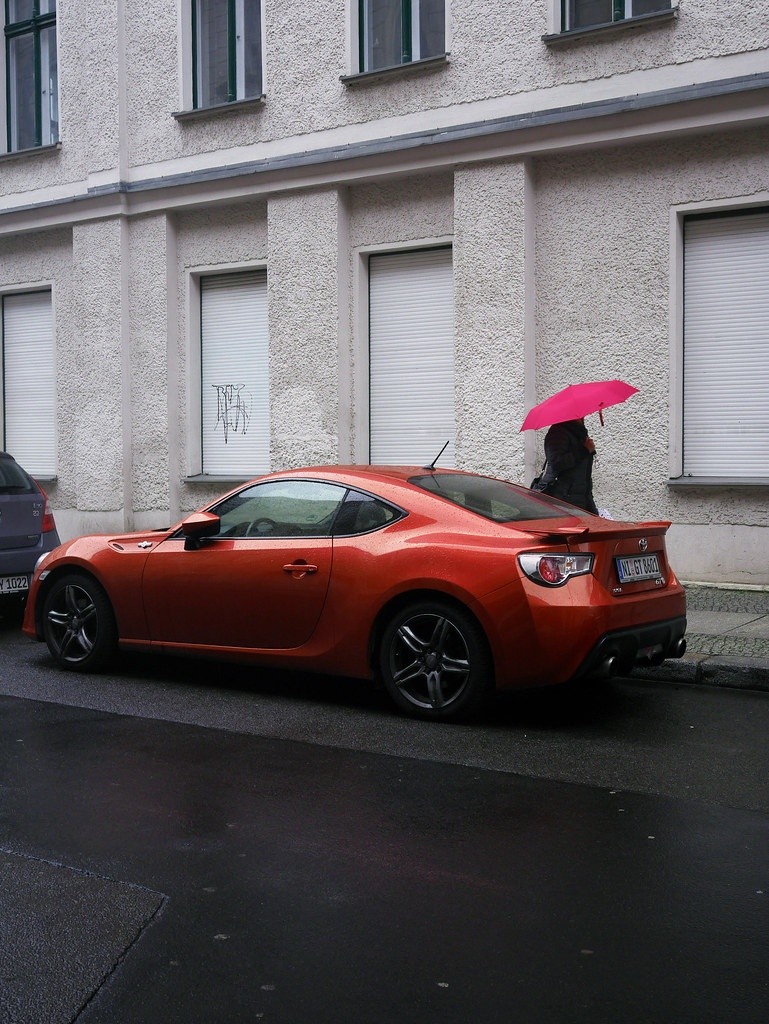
[0,451,60,608]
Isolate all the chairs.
[331,501,386,535]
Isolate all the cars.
[22,441,687,721]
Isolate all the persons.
[544,417,598,515]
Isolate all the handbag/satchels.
[529,459,560,496]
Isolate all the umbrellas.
[520,380,640,455]
